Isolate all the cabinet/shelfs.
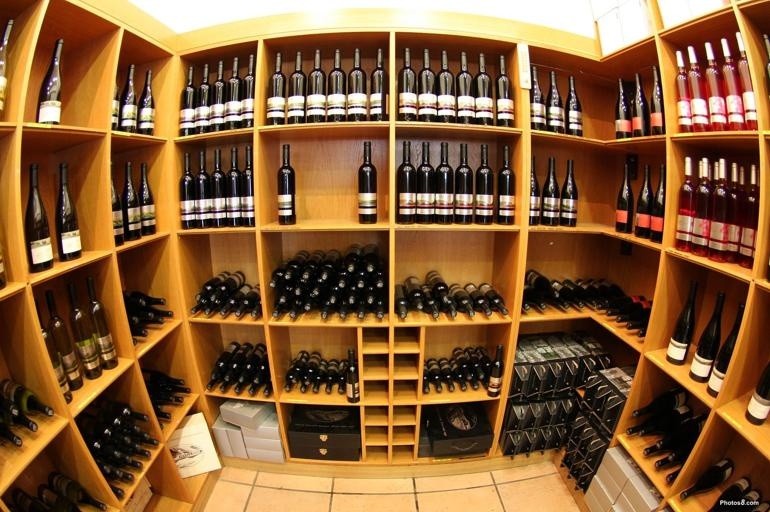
[0,0,226,512]
[553,0,770,512]
[173,8,600,481]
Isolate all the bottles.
[704,42,728,131]
[210,149,226,227]
[474,52,494,125]
[649,65,665,135]
[241,145,255,226]
[195,151,210,228]
[688,46,712,131]
[370,48,388,120]
[650,164,665,243]
[346,349,360,403]
[675,156,694,252]
[120,162,142,241]
[277,144,297,225]
[195,62,210,133]
[112,78,120,130]
[560,159,578,227]
[397,140,416,224]
[0,400,38,432]
[709,478,750,511]
[44,289,83,391]
[285,350,348,393]
[720,37,744,130]
[206,342,272,397]
[418,49,438,122]
[398,48,418,120]
[142,367,192,421]
[435,142,454,225]
[740,490,765,510]
[615,77,632,138]
[632,72,649,136]
[680,459,733,501]
[745,363,770,425]
[210,60,225,132]
[626,388,707,483]
[68,280,102,380]
[0,378,54,417]
[565,75,583,136]
[457,50,475,123]
[423,345,504,397]
[675,49,692,132]
[242,54,255,128]
[475,144,493,225]
[454,143,474,223]
[138,163,156,235]
[138,70,155,135]
[523,269,653,337]
[307,49,326,122]
[736,31,757,130]
[191,271,261,317]
[266,53,286,125]
[32,298,73,405]
[394,270,509,319]
[23,163,53,273]
[358,142,377,224]
[0,18,16,122]
[615,164,634,232]
[328,48,346,122]
[180,152,195,230]
[85,276,118,370]
[666,280,699,365]
[437,50,457,122]
[635,165,654,238]
[348,47,367,121]
[124,289,174,345]
[542,156,561,226]
[497,146,515,225]
[78,398,159,497]
[706,304,746,396]
[531,66,546,130]
[225,57,242,129]
[180,67,197,136]
[36,37,65,124]
[121,64,137,132]
[56,162,83,262]
[110,162,125,245]
[270,244,385,320]
[692,157,760,269]
[226,147,241,227]
[495,55,514,127]
[530,154,540,225]
[287,51,306,123]
[11,471,106,512]
[689,286,725,382]
[0,420,22,448]
[416,141,434,224]
[546,71,566,133]
